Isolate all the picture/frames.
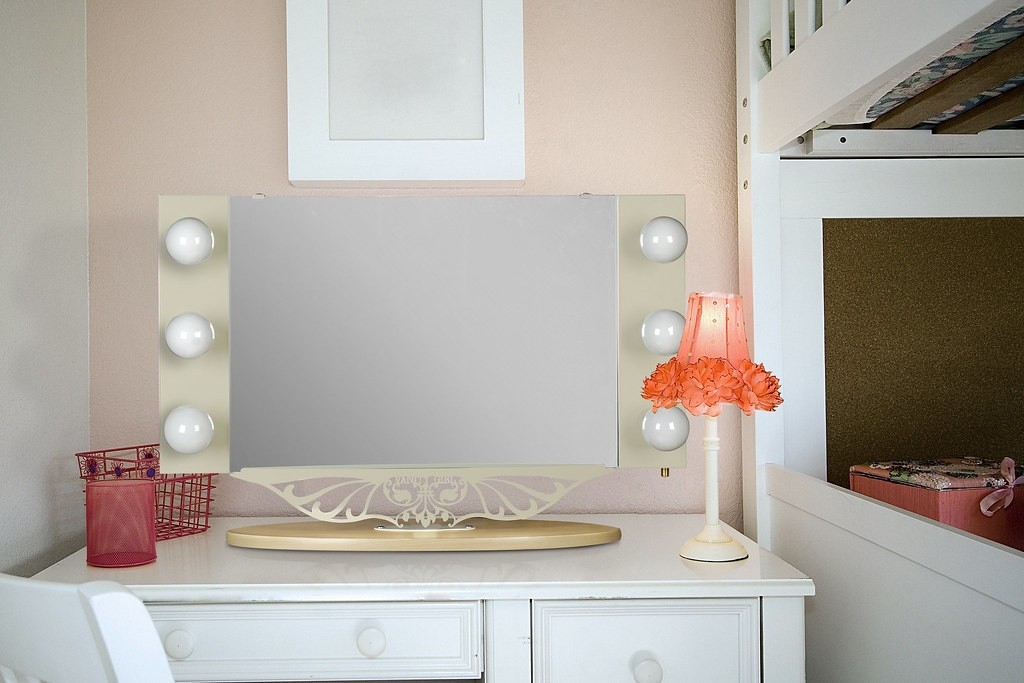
[285,0,525,191]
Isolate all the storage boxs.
[847,458,1023,545]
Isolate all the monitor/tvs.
[155,193,690,552]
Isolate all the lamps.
[641,292,784,563]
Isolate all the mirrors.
[228,195,618,468]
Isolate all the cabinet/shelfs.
[734,1,1024,683]
[30,513,816,683]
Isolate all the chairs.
[0,570,176,683]
